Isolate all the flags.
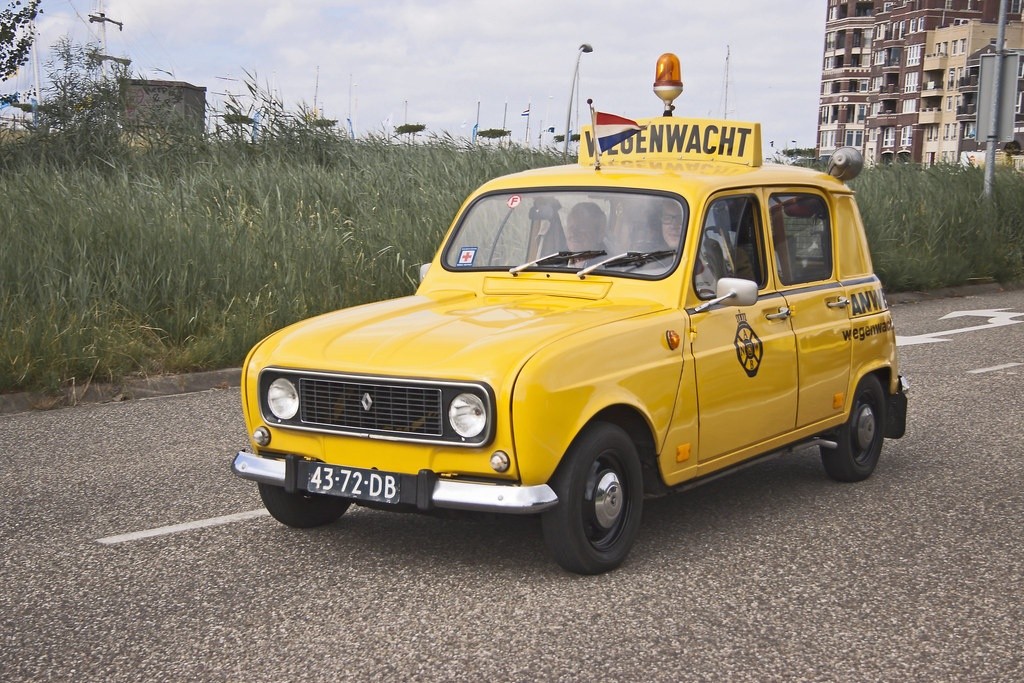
[521,109,529,116]
[548,126,554,133]
[593,110,647,155]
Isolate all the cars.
[227,49,909,577]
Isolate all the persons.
[566,202,718,297]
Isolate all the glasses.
[659,213,686,225]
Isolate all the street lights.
[561,42,594,157]
